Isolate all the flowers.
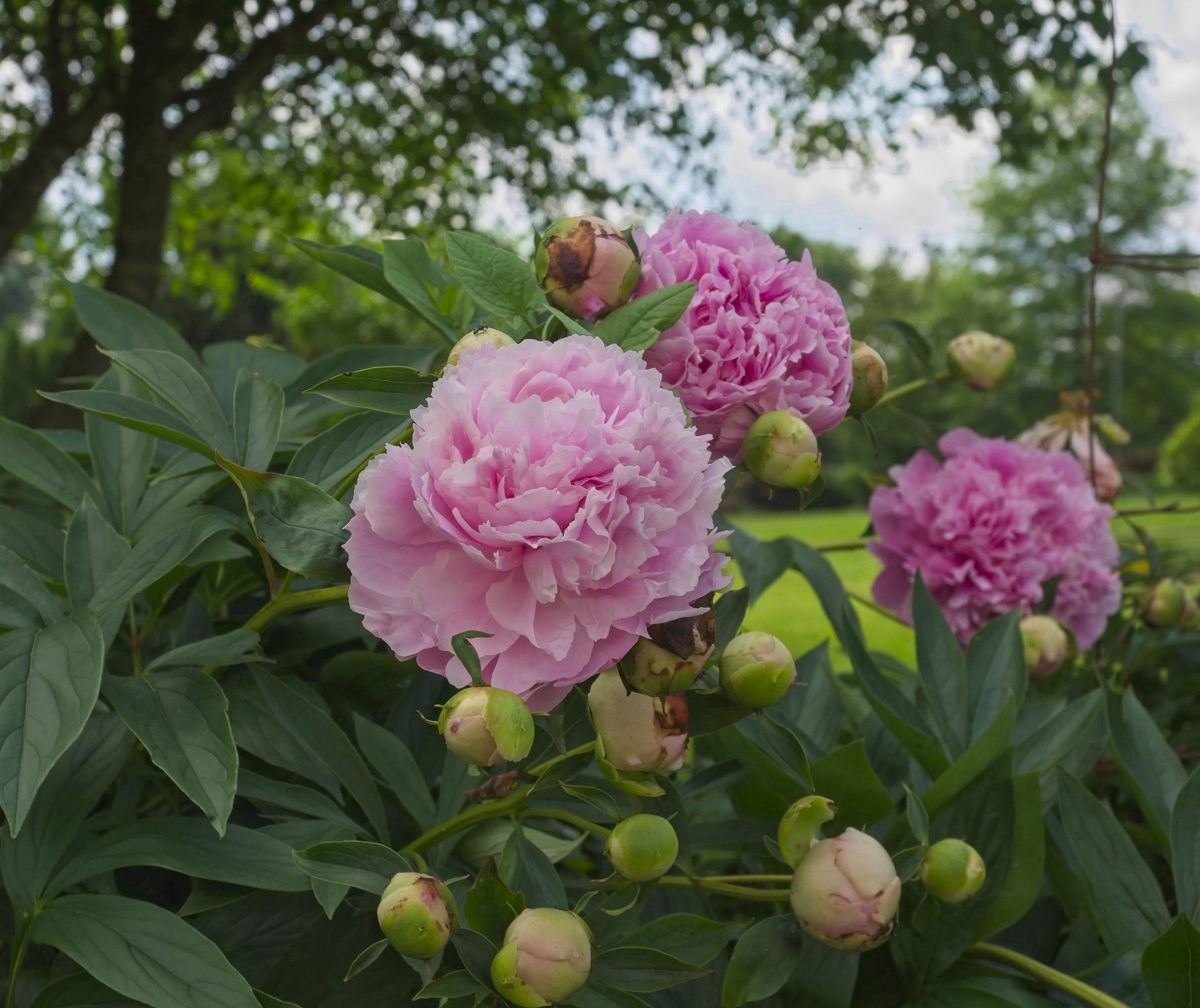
[868,424,1118,666]
[626,209,854,460]
[342,337,739,713]
